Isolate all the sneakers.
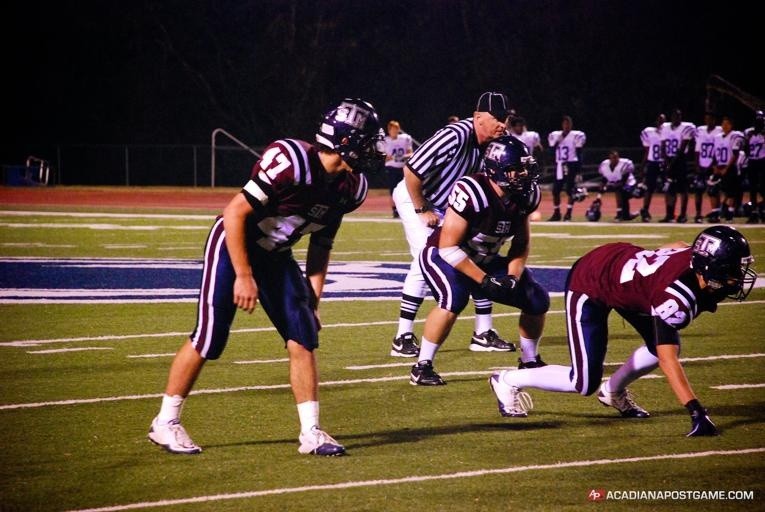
[147,417,203,455]
[488,355,548,417]
[597,380,650,418]
[299,430,345,456]
[409,360,447,386]
[389,332,420,358]
[471,328,517,352]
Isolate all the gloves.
[686,409,720,437]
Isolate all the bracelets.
[685,400,701,415]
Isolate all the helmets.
[314,98,386,174]
[481,136,539,204]
[691,226,757,301]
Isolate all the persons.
[390,92,516,358]
[381,120,413,217]
[490,226,757,438]
[504,109,765,224]
[408,135,549,387]
[448,116,459,124]
[148,97,379,457]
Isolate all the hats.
[478,91,521,124]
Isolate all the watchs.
[415,206,428,213]
